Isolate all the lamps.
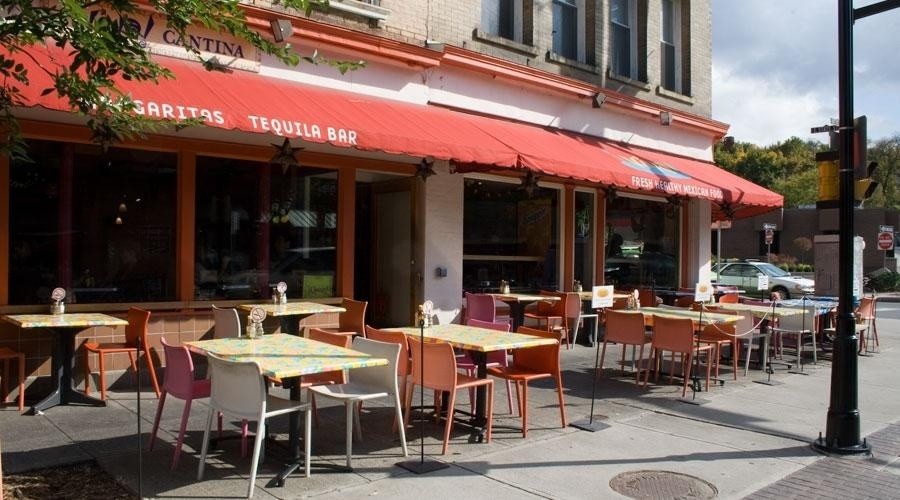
[269,16,294,45]
[659,111,674,128]
[592,90,609,109]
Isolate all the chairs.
[203,302,242,380]
[365,282,883,462]
[147,335,211,472]
[267,327,352,439]
[310,295,370,339]
[196,348,312,499]
[305,336,408,481]
[0,346,24,412]
[83,303,160,404]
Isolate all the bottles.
[625,293,635,310]
[573,279,578,293]
[499,278,506,294]
[415,304,428,327]
[49,299,66,314]
[246,317,256,339]
[271,287,279,304]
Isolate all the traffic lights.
[854,160,879,203]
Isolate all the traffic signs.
[762,223,777,230]
[879,225,894,233]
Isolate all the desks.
[182,338,390,466]
[4,313,130,417]
[238,300,348,390]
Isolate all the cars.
[216,250,334,299]
[710,261,815,302]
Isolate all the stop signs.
[878,232,894,251]
[765,230,774,244]
